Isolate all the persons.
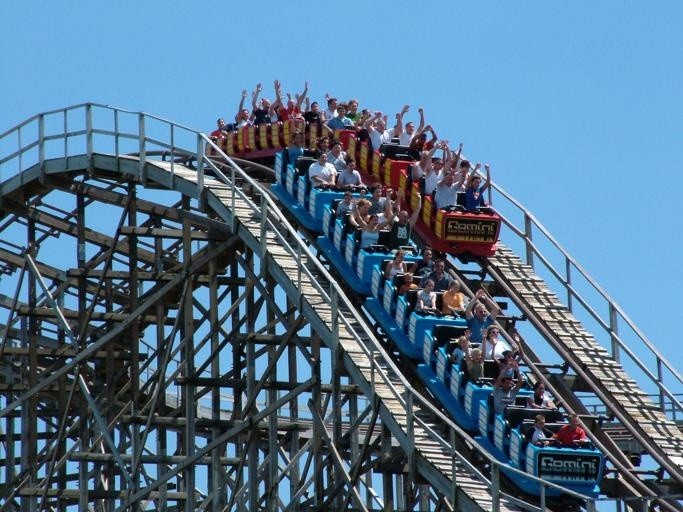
[524,382,558,411]
[357,104,491,211]
[490,357,522,413]
[549,414,587,447]
[288,130,422,248]
[354,227,525,383]
[525,415,558,447]
[211,80,363,133]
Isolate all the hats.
[487,324,499,331]
